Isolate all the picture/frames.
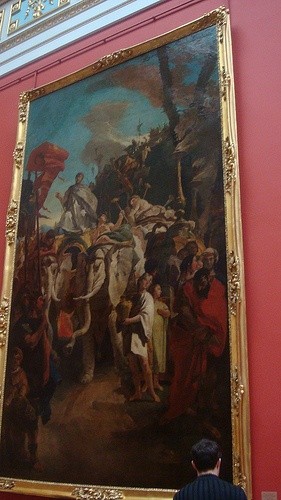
[0,5,253,500]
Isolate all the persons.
[170,437,248,500]
